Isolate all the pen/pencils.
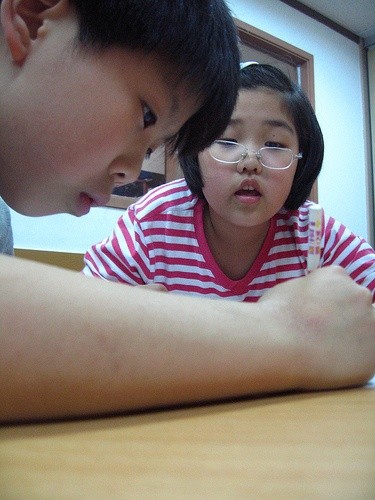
[306,203,325,275]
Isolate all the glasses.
[208,139,304,170]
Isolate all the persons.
[82,63,375,305]
[0,0,375,422]
